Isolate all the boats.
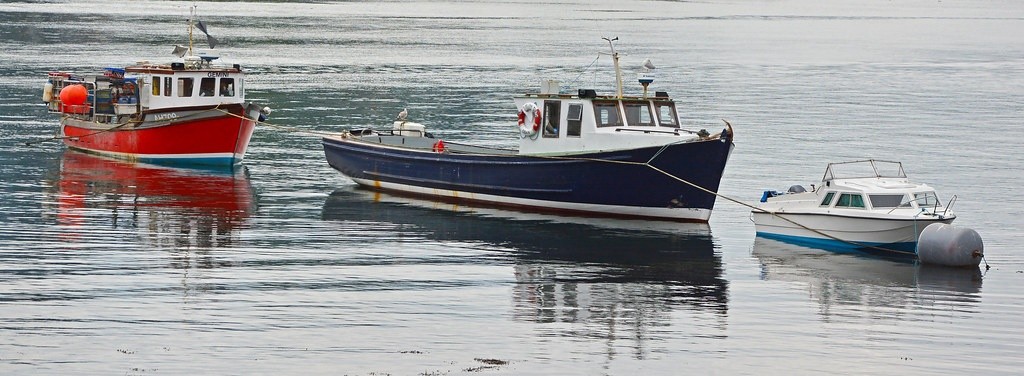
[43,4,265,170]
[753,157,959,254]
[324,34,734,223]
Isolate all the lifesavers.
[518,104,541,136]
